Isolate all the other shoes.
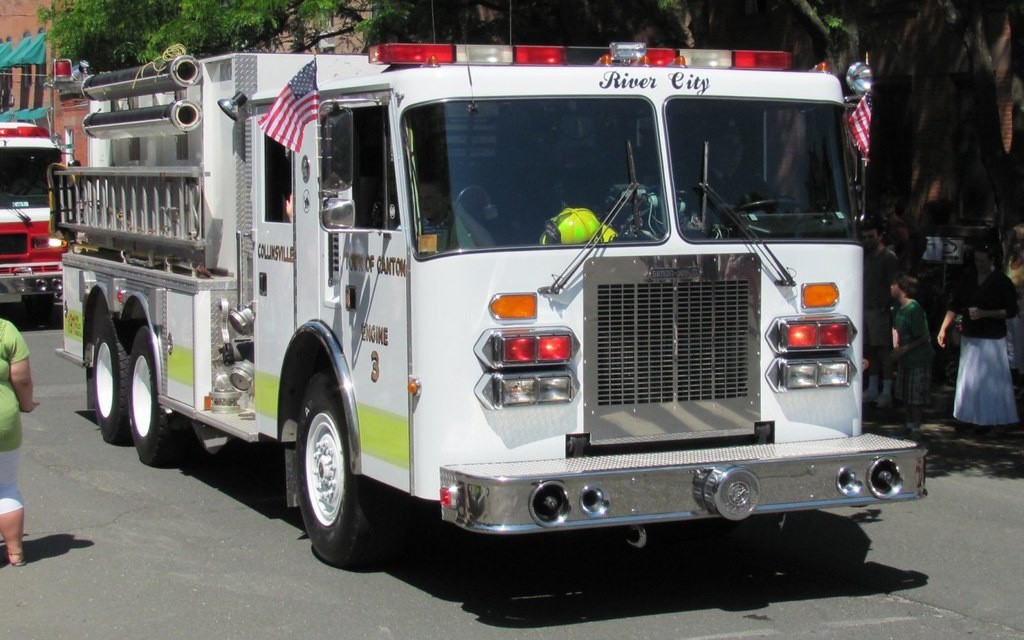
[964,426,982,435]
[895,427,911,435]
[911,430,922,441]
[986,428,1007,438]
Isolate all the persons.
[889,271,935,436]
[396,181,496,252]
[937,226,1024,437]
[1,317,40,566]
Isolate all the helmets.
[539,207,618,245]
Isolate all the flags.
[848,89,872,167]
[257,58,320,153]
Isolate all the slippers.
[14,560,26,566]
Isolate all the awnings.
[0,107,50,122]
[0,33,46,71]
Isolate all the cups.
[969,307,978,320]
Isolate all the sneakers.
[873,393,894,408]
[862,390,880,403]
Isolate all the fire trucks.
[45,41,930,574]
[0,120,84,329]
